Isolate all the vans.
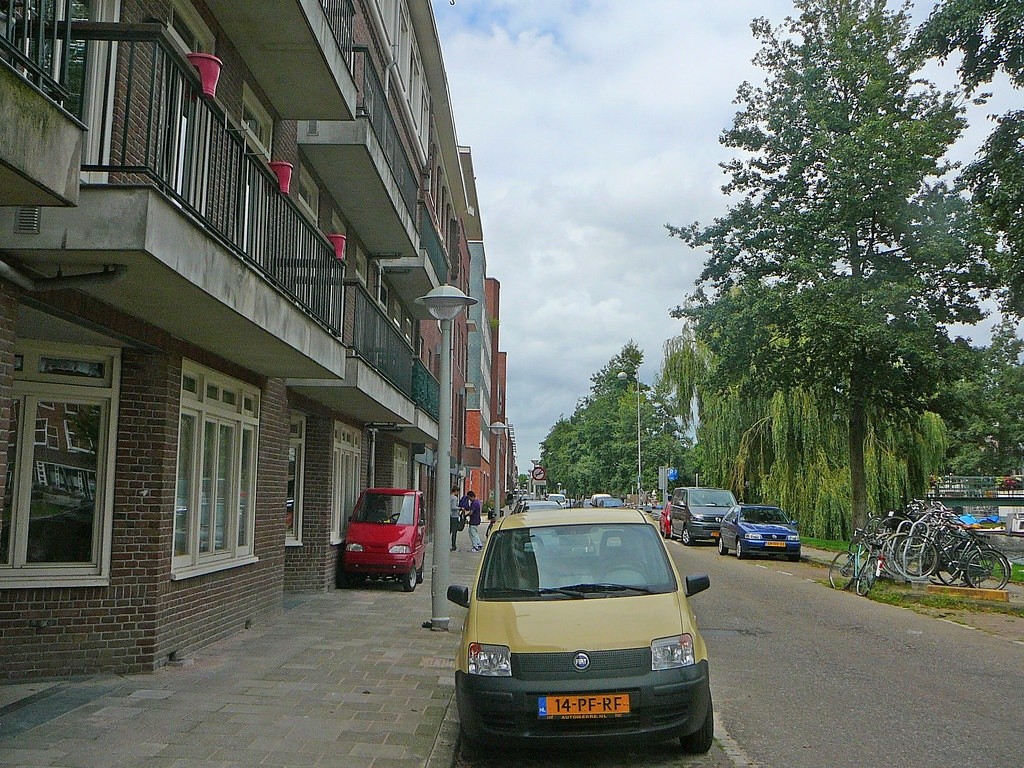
[668,487,740,546]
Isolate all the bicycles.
[828,495,1013,597]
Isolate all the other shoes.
[451,547,460,552]
[467,546,479,552]
[477,545,483,551]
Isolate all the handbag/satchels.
[457,514,466,531]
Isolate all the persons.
[507,491,513,510]
[929,472,938,490]
[449,484,465,550]
[465,490,484,552]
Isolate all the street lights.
[416,281,479,633]
[488,421,508,520]
[617,359,643,507]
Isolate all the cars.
[512,488,626,512]
[718,504,801,559]
[344,488,427,591]
[658,502,672,538]
[446,507,715,767]
